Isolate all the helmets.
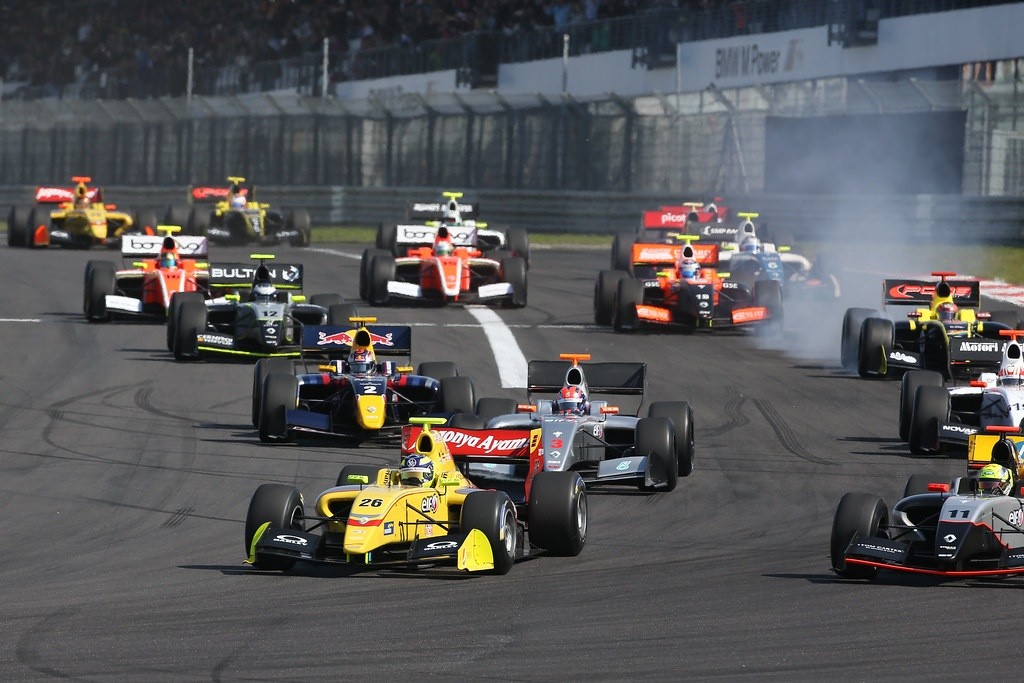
[436,241,454,256]
[555,386,585,416]
[678,259,701,281]
[158,251,180,272]
[228,195,246,212]
[72,196,92,211]
[936,302,959,323]
[254,283,278,306]
[397,453,435,486]
[348,348,374,376]
[998,364,1024,387]
[979,463,1013,495]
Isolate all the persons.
[229,193,247,208]
[74,197,92,209]
[935,302,961,321]
[435,241,454,256]
[554,385,589,416]
[159,251,175,267]
[996,365,1024,388]
[676,259,701,280]
[739,235,760,254]
[252,283,278,299]
[441,212,456,224]
[974,464,1013,497]
[399,454,433,485]
[347,348,375,372]
